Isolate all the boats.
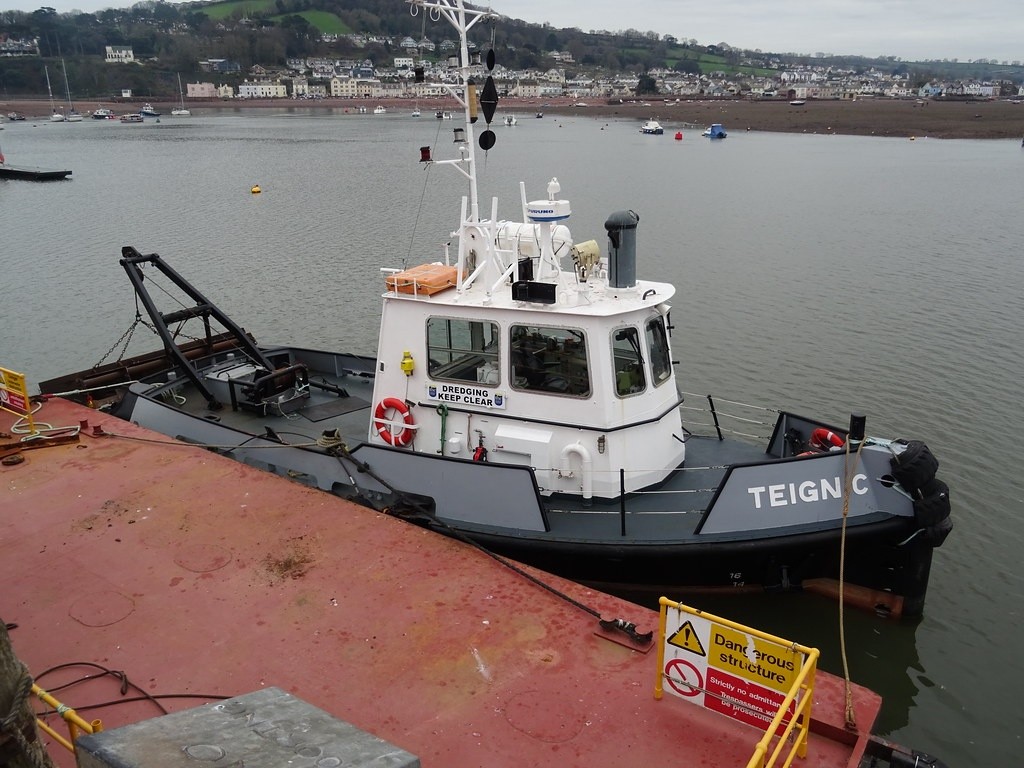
[535,110,544,118]
[641,117,663,134]
[789,99,806,105]
[93,107,115,120]
[702,123,727,138]
[252,184,261,193]
[442,110,452,120]
[374,105,386,113]
[576,103,587,107]
[140,103,161,117]
[0,162,72,182]
[435,109,443,118]
[411,107,421,117]
[504,114,518,125]
[120,113,144,123]
[358,106,367,112]
[171,71,190,117]
[34,0,957,582]
[9,112,26,120]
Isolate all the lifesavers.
[809,428,845,452]
[375,397,415,447]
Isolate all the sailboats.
[60,57,83,121]
[43,65,65,121]
